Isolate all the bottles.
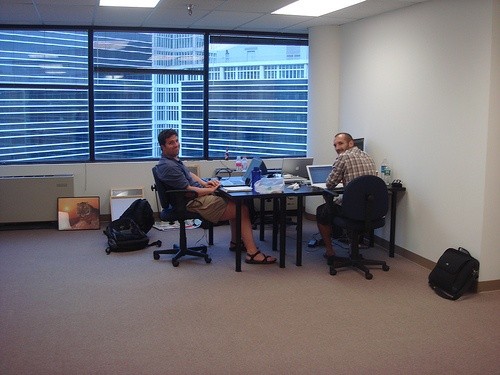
[241,155,248,172]
[381,158,390,181]
[385,164,391,185]
[235,156,242,172]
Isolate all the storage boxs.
[111,189,143,222]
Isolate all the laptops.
[281,158,313,178]
[218,158,262,187]
[306,165,344,189]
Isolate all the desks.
[202,175,407,271]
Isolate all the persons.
[155,129,278,264]
[316,132,377,264]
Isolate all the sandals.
[245,251,277,264]
[229,241,259,252]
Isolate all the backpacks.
[103,218,162,255]
[119,198,154,233]
[428,247,480,300]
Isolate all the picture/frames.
[57,196,100,231]
[185,164,200,177]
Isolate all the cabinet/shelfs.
[217,169,297,217]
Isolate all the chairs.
[153,190,211,267]
[322,175,389,280]
